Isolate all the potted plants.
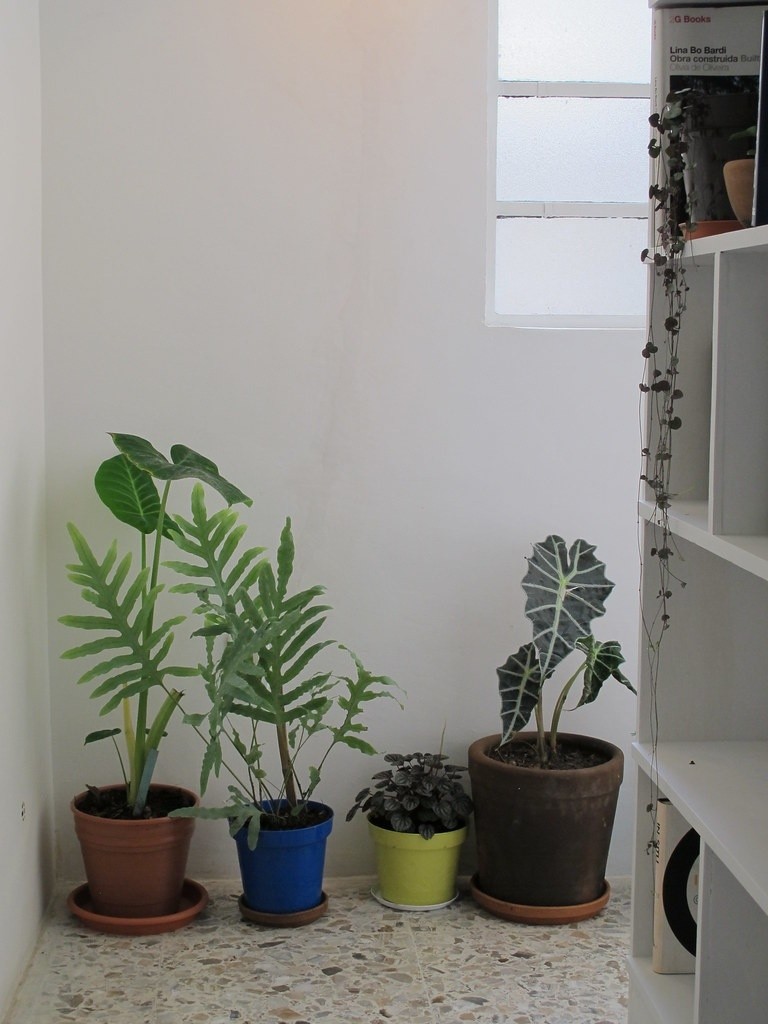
[463,534,637,926]
[722,126,765,227]
[57,480,409,928]
[631,74,757,897]
[64,431,257,935]
[345,753,470,911]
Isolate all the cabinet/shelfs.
[627,226,768,1024]
